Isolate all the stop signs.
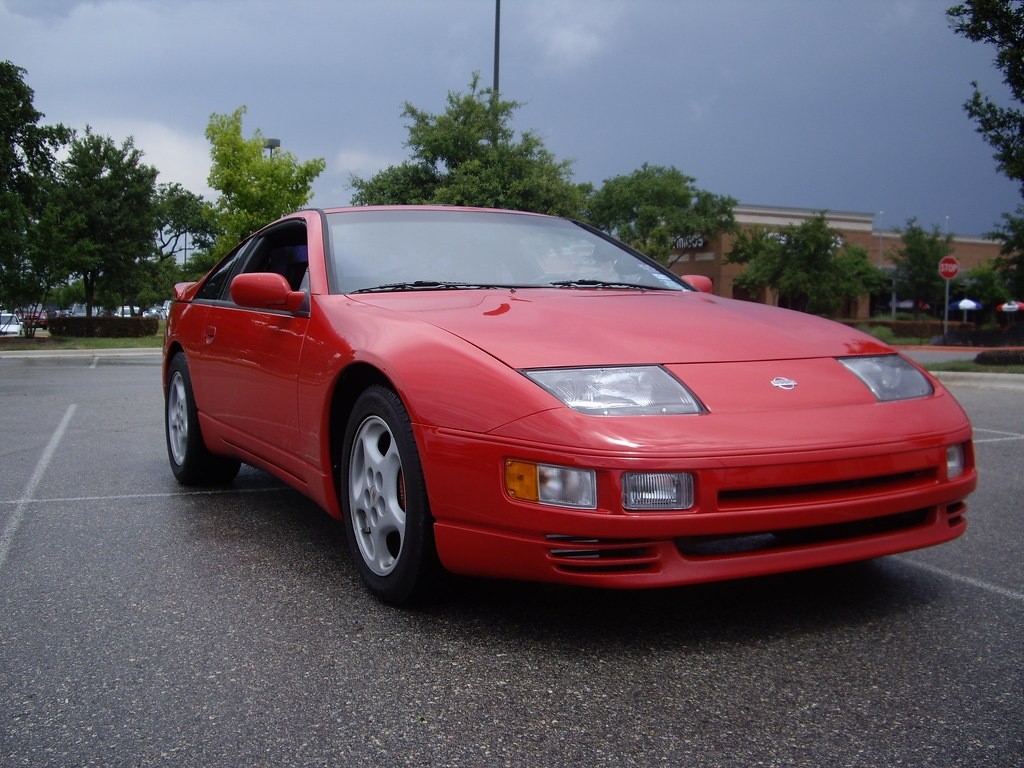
[937,258,959,281]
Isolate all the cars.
[163,200,978,606]
[60,310,72,318]
[0,313,24,336]
[147,307,166,321]
[115,306,146,318]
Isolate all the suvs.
[15,303,48,330]
[163,301,171,318]
[71,302,99,316]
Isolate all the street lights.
[946,214,950,257]
[879,209,884,269]
